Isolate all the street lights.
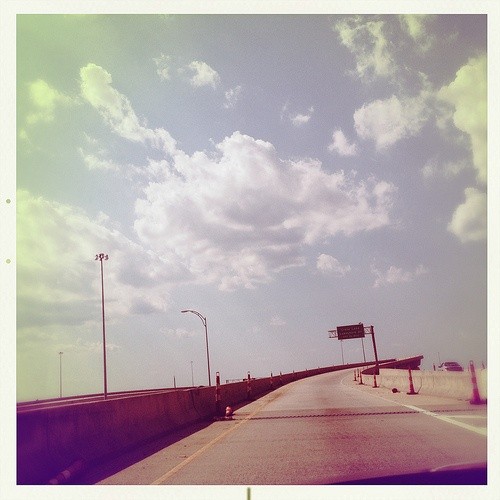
[181,309,212,386]
[95,253,109,400]
[359,321,366,362]
[58,351,64,398]
[190,361,195,386]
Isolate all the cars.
[437,361,462,372]
